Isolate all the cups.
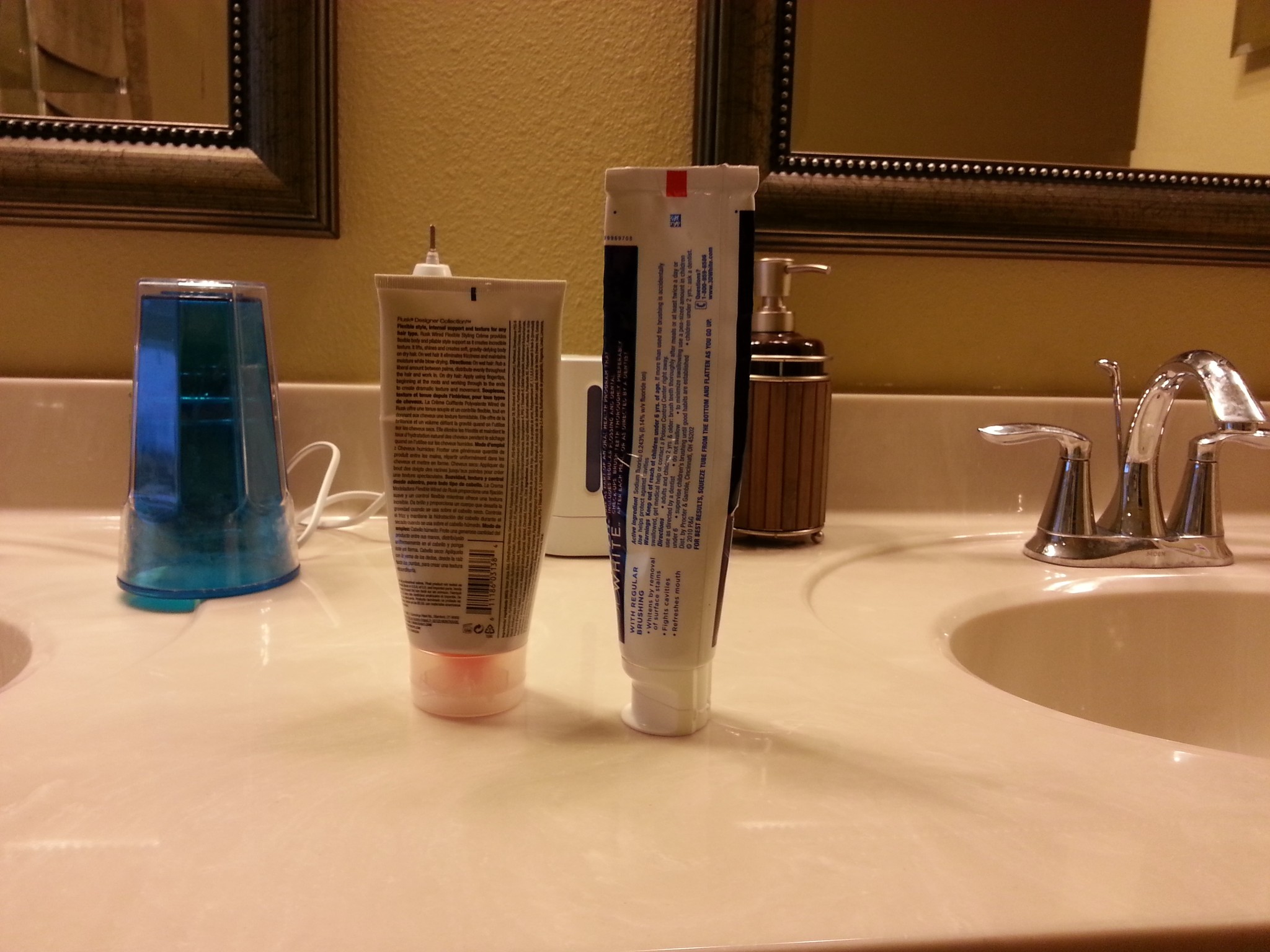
[106,270,301,601]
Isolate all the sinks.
[930,578,1268,769]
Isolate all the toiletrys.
[369,274,571,720]
[597,163,762,740]
[726,254,837,547]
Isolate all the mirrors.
[0,0,342,240]
[691,1,1270,268]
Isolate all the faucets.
[976,350,1270,568]
